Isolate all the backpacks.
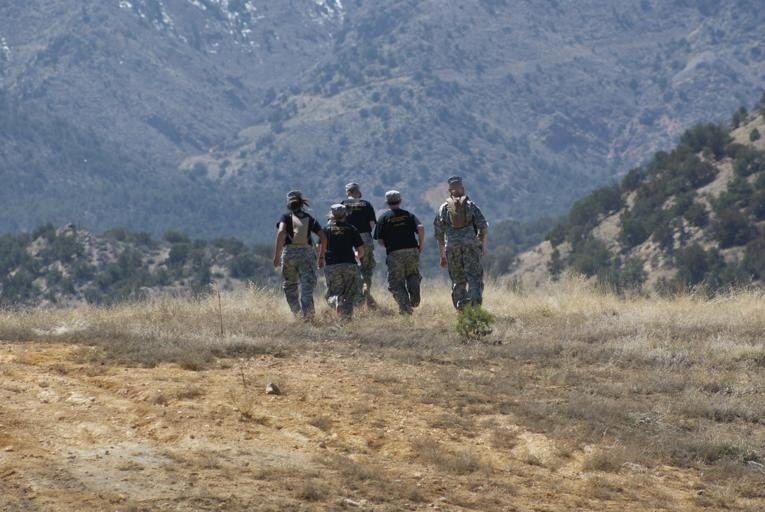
[446,195,471,230]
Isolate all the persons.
[372,190,425,317]
[314,203,365,329]
[430,174,489,319]
[338,179,379,317]
[272,190,329,321]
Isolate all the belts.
[284,244,312,249]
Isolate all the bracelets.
[319,256,326,259]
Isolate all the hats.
[384,189,401,203]
[286,189,303,204]
[345,182,362,198]
[330,203,347,214]
[447,175,464,185]
